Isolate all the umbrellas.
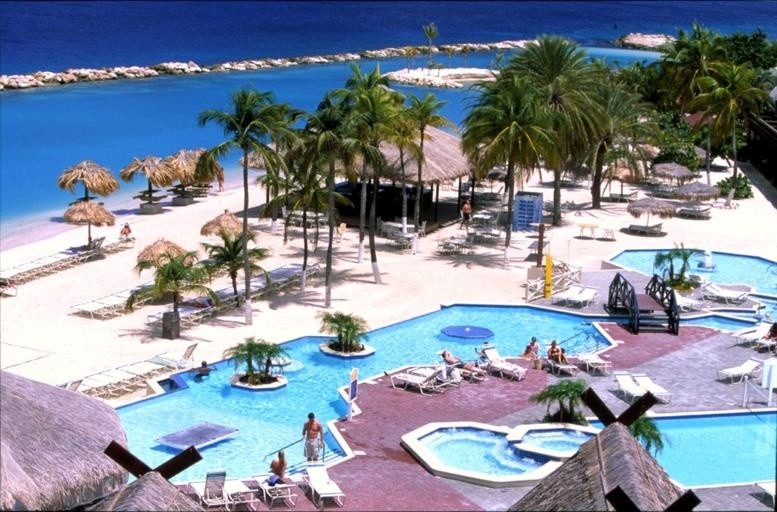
[63,200,116,246]
[58,159,118,201]
[138,238,194,272]
[199,208,258,247]
[601,144,721,230]
[121,149,217,203]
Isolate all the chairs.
[46,351,201,402]
[385,343,614,397]
[219,477,262,510]
[615,370,648,406]
[0,236,140,289]
[185,469,229,510]
[632,372,674,404]
[253,472,300,510]
[302,459,347,509]
[550,283,600,309]
[69,247,329,337]
[713,315,775,396]
[664,270,752,311]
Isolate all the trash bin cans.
[163,312,181,340]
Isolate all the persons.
[528,336,540,354]
[217,168,224,192]
[548,340,571,365]
[758,323,777,348]
[443,350,486,376]
[523,345,536,359]
[459,199,472,232]
[269,451,288,478]
[302,412,324,461]
